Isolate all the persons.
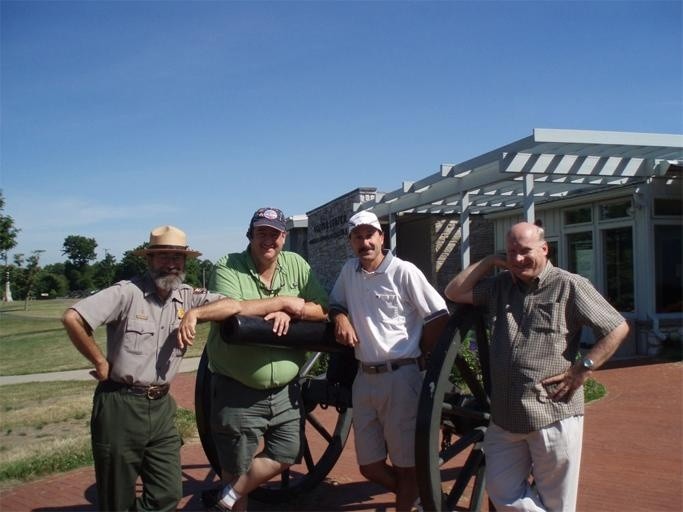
[195,207,329,512]
[325,210,451,512]
[62,225,242,512]
[444,222,629,512]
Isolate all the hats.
[133,226,202,258]
[348,211,381,236]
[253,207,287,232]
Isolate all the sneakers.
[201,487,234,512]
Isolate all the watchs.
[580,355,594,373]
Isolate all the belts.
[361,358,415,374]
[112,382,171,401]
